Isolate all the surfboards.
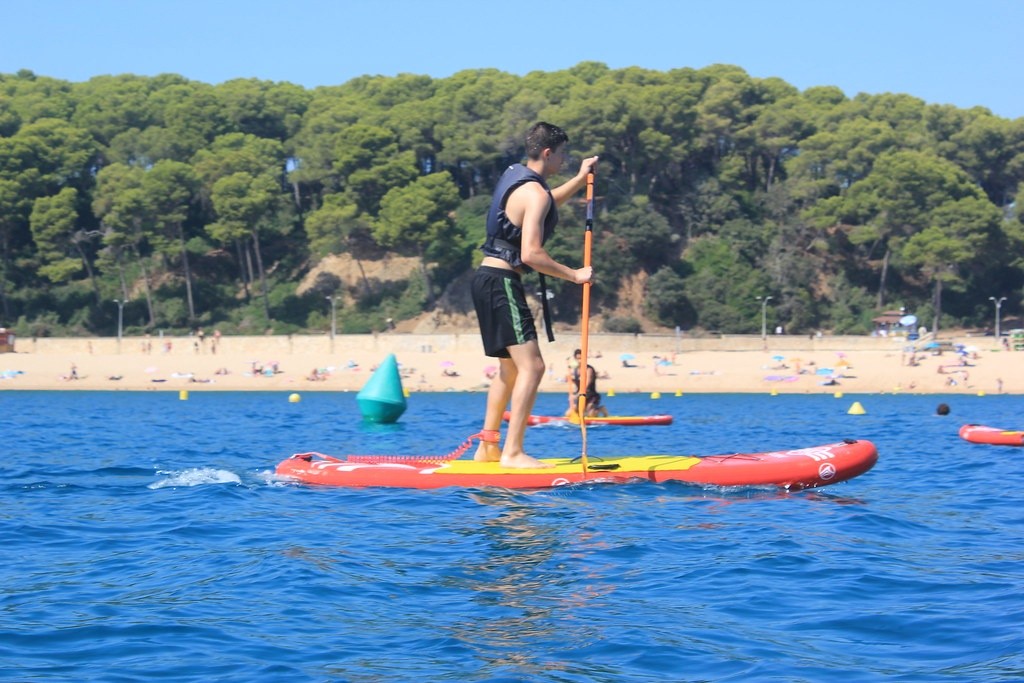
[273,437,881,491]
[958,423,1024,447]
[501,408,673,427]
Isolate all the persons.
[252,361,278,377]
[410,368,497,387]
[308,368,325,380]
[562,349,607,417]
[472,122,598,469]
[71,363,77,379]
[623,336,1010,387]
[216,331,220,342]
[195,341,198,350]
[211,339,215,353]
[198,330,204,341]
[140,340,151,353]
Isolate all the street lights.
[112,297,129,338]
[988,295,1007,341]
[325,295,342,340]
[755,295,775,342]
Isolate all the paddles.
[578,164,595,478]
[563,358,582,423]
